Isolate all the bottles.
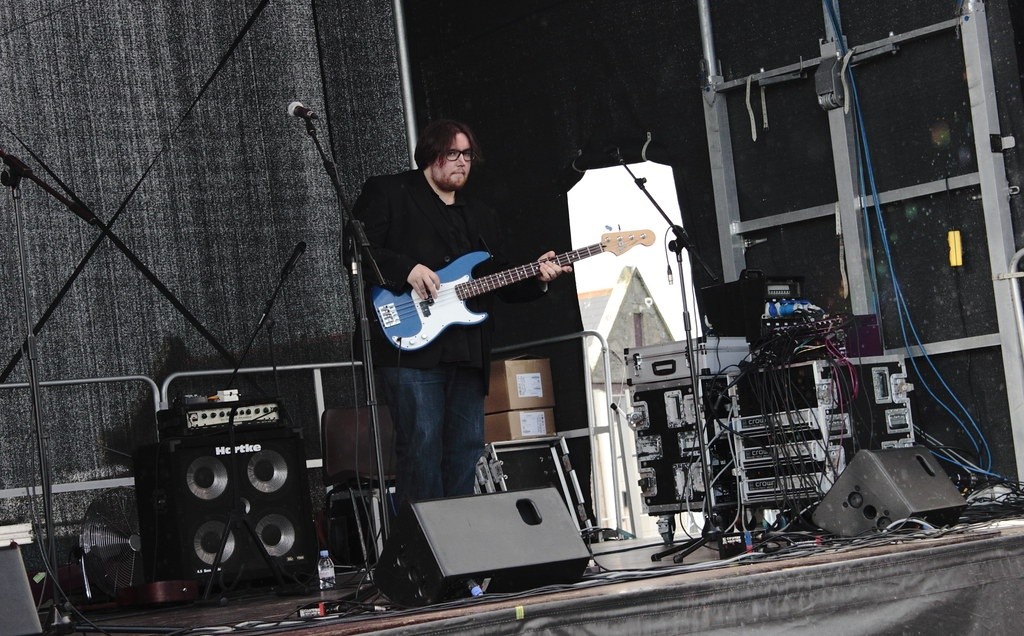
[318,550,335,590]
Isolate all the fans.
[78,487,145,594]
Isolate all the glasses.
[445,150,477,162]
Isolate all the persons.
[338,118,572,516]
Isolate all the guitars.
[368,223,656,353]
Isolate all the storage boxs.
[483,408,556,442]
[483,358,556,415]
[624,337,750,387]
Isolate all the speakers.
[813,444,969,537]
[0,544,43,636]
[372,486,591,611]
[134,422,322,581]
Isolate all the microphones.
[288,101,319,119]
[282,241,307,276]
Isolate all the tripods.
[612,152,772,565]
[202,404,292,603]
